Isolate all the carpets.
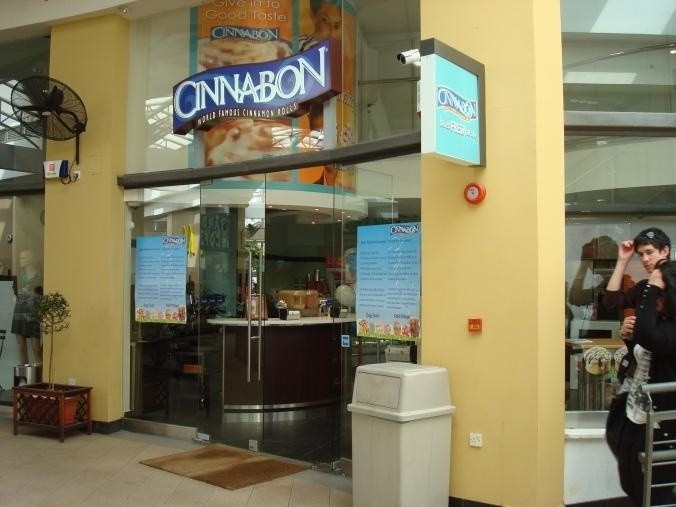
[138,442,310,491]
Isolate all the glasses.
[640,231,656,238]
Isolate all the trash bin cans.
[13,364,42,420]
[346,361,456,507]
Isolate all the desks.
[566,335,627,410]
[569,316,624,390]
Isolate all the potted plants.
[11,288,95,441]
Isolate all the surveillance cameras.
[397,48,421,65]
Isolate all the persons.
[11,248,43,365]
[603,227,670,372]
[161,270,217,379]
[569,232,640,360]
[616,258,676,504]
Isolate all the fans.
[9,74,88,164]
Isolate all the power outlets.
[468,431,482,448]
[72,170,80,178]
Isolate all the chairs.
[158,337,223,420]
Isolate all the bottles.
[279,301,287,319]
[313,268,321,287]
